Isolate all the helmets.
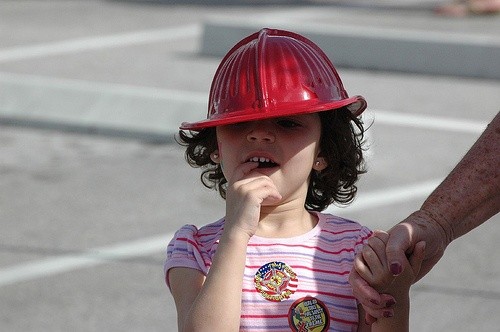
[178,28,368,133]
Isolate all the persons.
[349,111,500,323]
[165,28,426,332]
